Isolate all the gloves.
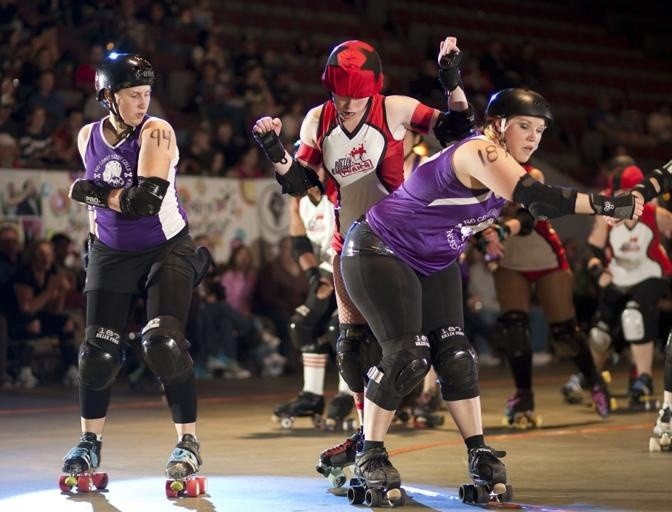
[302,267,325,298]
[591,264,609,288]
[497,226,512,242]
[251,129,288,165]
[591,192,635,222]
[71,179,112,212]
[439,50,464,94]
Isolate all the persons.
[54,50,211,500]
[113,0,310,178]
[582,90,671,166]
[0,226,23,279]
[392,124,451,435]
[2,242,80,388]
[48,233,84,317]
[252,235,316,373]
[186,236,279,381]
[270,140,395,433]
[176,340,236,380]
[339,88,645,507]
[600,160,672,452]
[457,267,552,374]
[562,165,672,412]
[251,37,479,489]
[0,0,112,169]
[212,244,288,380]
[415,33,549,166]
[473,163,617,432]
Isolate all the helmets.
[484,88,556,125]
[95,52,155,100]
[320,41,383,98]
[607,164,644,189]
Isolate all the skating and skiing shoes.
[506,390,537,430]
[590,381,615,420]
[648,402,672,455]
[167,434,208,496]
[562,377,586,407]
[60,434,109,493]
[459,446,514,504]
[629,376,655,413]
[347,446,406,507]
[393,385,446,427]
[275,392,324,429]
[321,394,358,433]
[317,425,365,488]
